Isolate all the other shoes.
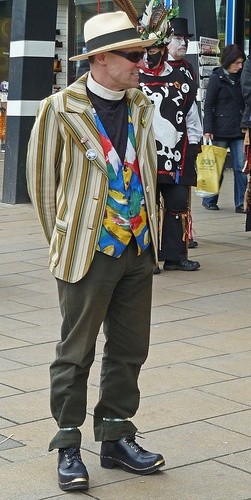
[188,241,198,247]
[154,266,160,274]
[235,205,246,213]
[163,259,200,271]
[202,202,219,210]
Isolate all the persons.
[161,18,202,247]
[202,44,246,212]
[240,54,251,231]
[136,17,203,274]
[26,10,166,491]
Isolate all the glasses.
[107,50,145,62]
[173,36,189,43]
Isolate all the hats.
[68,10,159,61]
[136,0,179,48]
[169,18,195,38]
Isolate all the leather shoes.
[57,448,89,491]
[100,437,165,474]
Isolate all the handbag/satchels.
[194,135,227,198]
